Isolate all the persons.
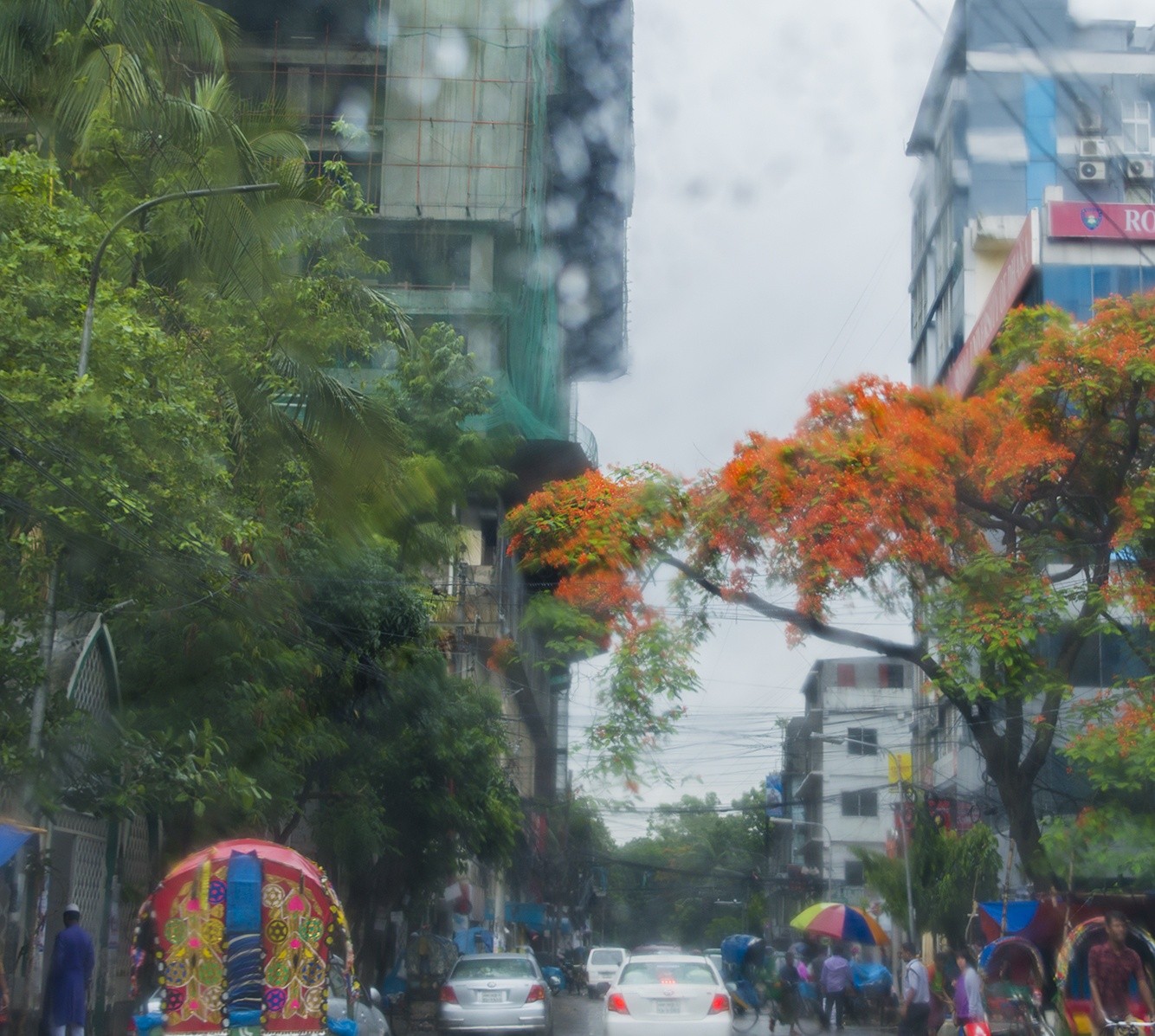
[45,902,96,1036]
[1085,906,1155,1035]
[767,947,993,1036]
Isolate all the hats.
[64,903,79,912]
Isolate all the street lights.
[768,816,834,904]
[809,729,917,947]
[22,182,288,823]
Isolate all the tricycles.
[973,936,1048,1036]
[720,934,830,1036]
[1051,910,1155,1036]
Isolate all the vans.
[583,947,632,999]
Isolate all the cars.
[433,952,553,1035]
[632,943,724,981]
[601,953,734,1036]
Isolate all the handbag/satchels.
[963,1017,992,1036]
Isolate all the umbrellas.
[788,901,890,949]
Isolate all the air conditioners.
[1127,159,1154,179]
[1079,113,1101,129]
[1080,139,1106,158]
[1078,161,1106,180]
